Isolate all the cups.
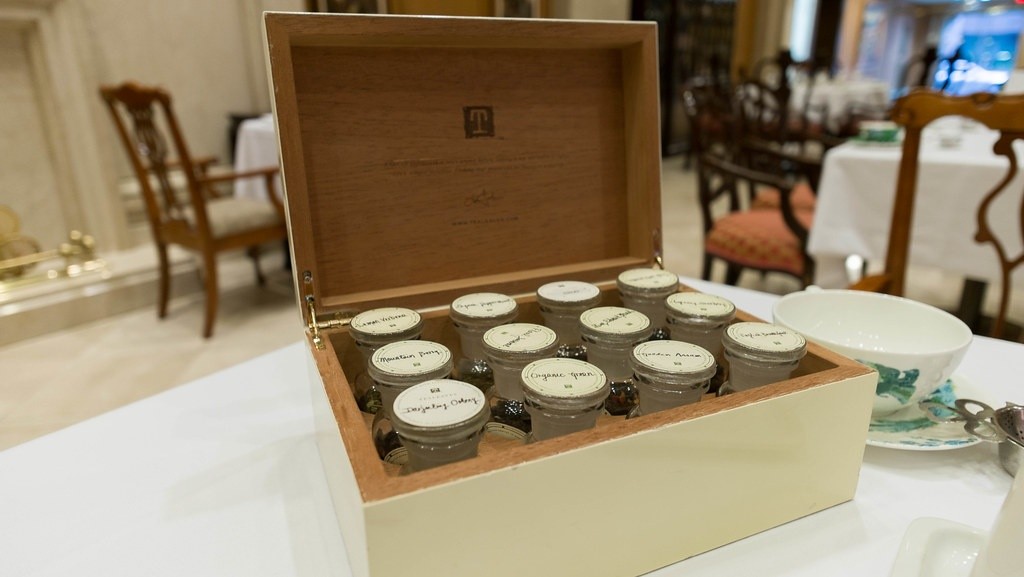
[858,121,898,141]
[772,285,973,415]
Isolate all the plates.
[888,517,990,577]
[865,372,1000,451]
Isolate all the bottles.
[618,268,679,342]
[454,292,519,393]
[520,357,610,442]
[369,340,455,465]
[391,378,490,471]
[489,322,560,433]
[535,280,602,362]
[349,307,424,413]
[721,321,807,391]
[578,306,654,415]
[626,340,717,420]
[664,292,736,393]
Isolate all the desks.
[805,113,1024,333]
[0,273,1024,577]
[743,73,895,161]
[230,111,295,290]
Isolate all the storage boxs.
[263,9,880,577]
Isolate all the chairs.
[95,43,1024,348]
